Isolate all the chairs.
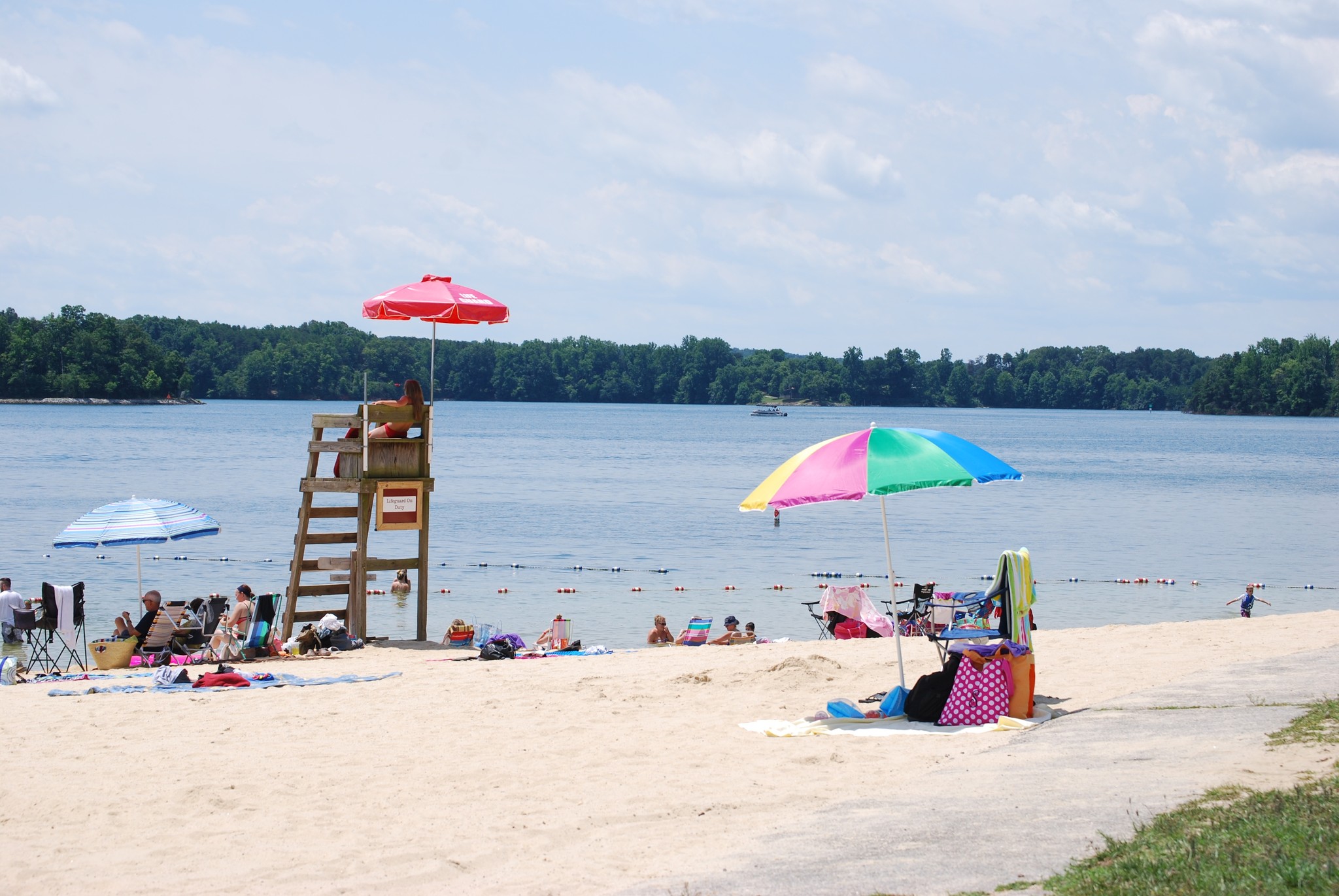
[113,599,203,668]
[441,625,474,646]
[666,616,713,647]
[800,547,1037,671]
[729,631,755,645]
[531,619,574,650]
[171,596,230,665]
[221,593,282,661]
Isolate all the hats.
[724,616,740,626]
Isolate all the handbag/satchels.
[834,622,867,640]
[902,652,963,722]
[0,656,18,685]
[294,625,317,646]
[478,638,516,661]
[549,638,568,650]
[486,633,527,651]
[963,644,1036,720]
[471,615,502,648]
[315,627,347,652]
[330,633,352,651]
[937,656,1014,726]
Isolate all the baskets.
[88,637,139,671]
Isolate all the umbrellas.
[739,419,1023,697]
[361,274,509,405]
[53,496,222,626]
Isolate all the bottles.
[349,635,356,641]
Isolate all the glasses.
[659,623,666,625]
[142,599,153,604]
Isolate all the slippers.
[328,646,342,654]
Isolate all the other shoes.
[305,649,316,657]
[318,648,331,655]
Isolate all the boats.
[750,409,787,417]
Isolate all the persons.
[675,616,705,644]
[531,627,553,650]
[708,616,742,645]
[367,380,426,438]
[647,615,674,644]
[1227,585,1271,617]
[451,619,465,625]
[204,584,257,660]
[167,597,206,655]
[23,599,32,610]
[390,569,411,594]
[743,622,757,643]
[113,590,162,654]
[0,577,27,643]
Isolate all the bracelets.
[667,632,670,634]
[126,620,132,624]
[543,631,546,634]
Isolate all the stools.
[10,579,88,675]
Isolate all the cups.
[291,642,299,656]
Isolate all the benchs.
[338,403,433,478]
